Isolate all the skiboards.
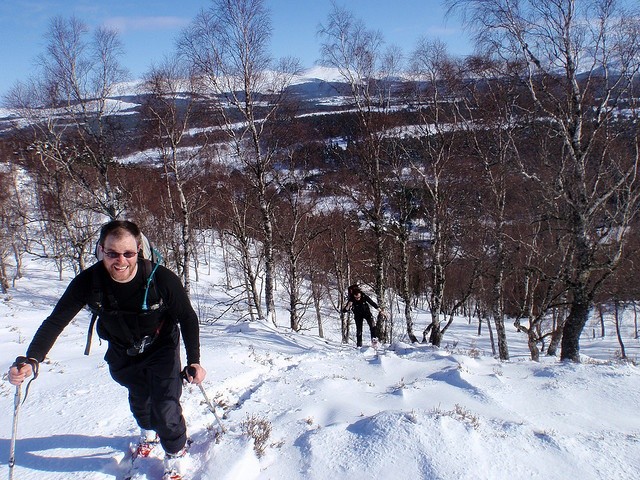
[116,435,193,480]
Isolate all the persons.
[8,220,206,480]
[342,289,382,350]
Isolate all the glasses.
[100,247,138,259]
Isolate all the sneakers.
[161,445,188,480]
[131,432,161,463]
